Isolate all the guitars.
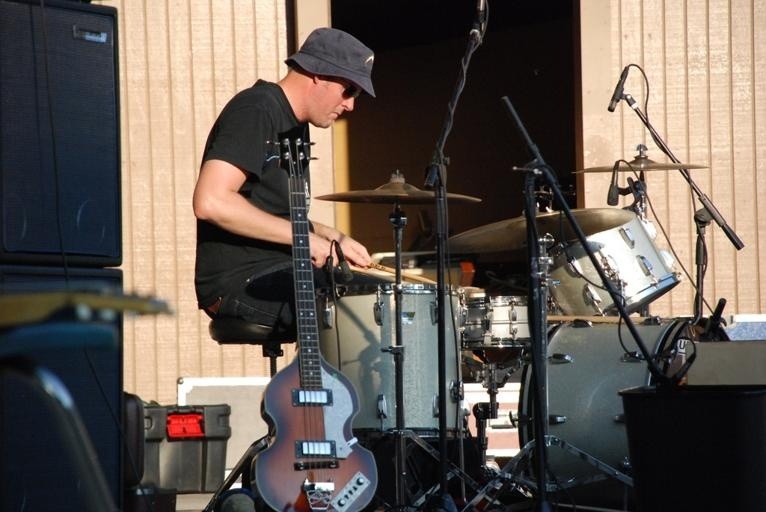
[249,134,379,512]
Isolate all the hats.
[285,28,376,98]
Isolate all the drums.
[542,208,679,318]
[519,316,733,487]
[319,280,464,441]
[460,297,532,348]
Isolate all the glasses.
[342,78,356,99]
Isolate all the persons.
[192,25,381,330]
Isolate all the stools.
[202,317,296,511]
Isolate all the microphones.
[607,161,619,205]
[663,352,696,388]
[334,241,354,282]
[607,66,629,112]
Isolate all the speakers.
[0,0,123,268]
[0,268,124,510]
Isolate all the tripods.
[461,234,632,512]
[366,201,501,512]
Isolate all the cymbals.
[569,159,706,172]
[444,208,636,253]
[313,181,481,205]
